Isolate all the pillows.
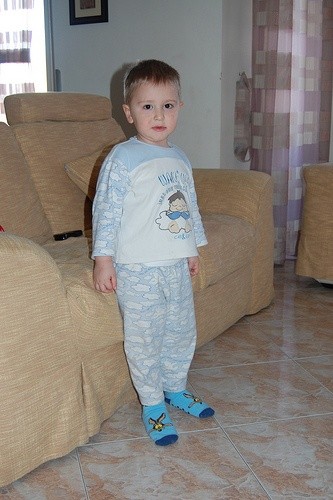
[65,135,128,203]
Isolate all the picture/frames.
[69,0,108,25]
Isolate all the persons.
[91,59,215,447]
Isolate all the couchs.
[296,161,333,289]
[0,93,274,488]
